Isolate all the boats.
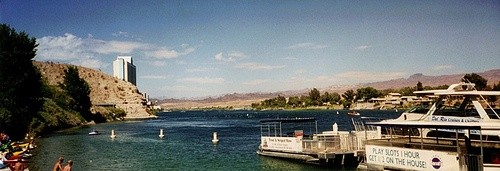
[89,131,102,135]
[363,81,500,138]
[0,141,28,169]
[347,112,360,116]
[260,116,317,123]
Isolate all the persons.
[0,131,38,171]
[53,156,64,171]
[61,160,73,171]
[91,126,96,132]
